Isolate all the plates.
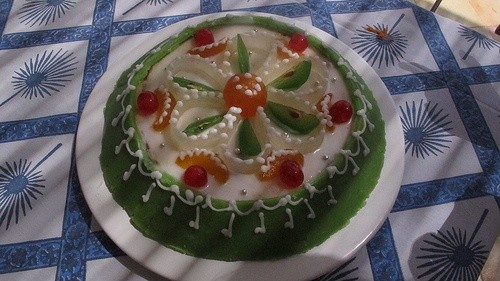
[75,10,406,281]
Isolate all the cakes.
[98,13,386,262]
